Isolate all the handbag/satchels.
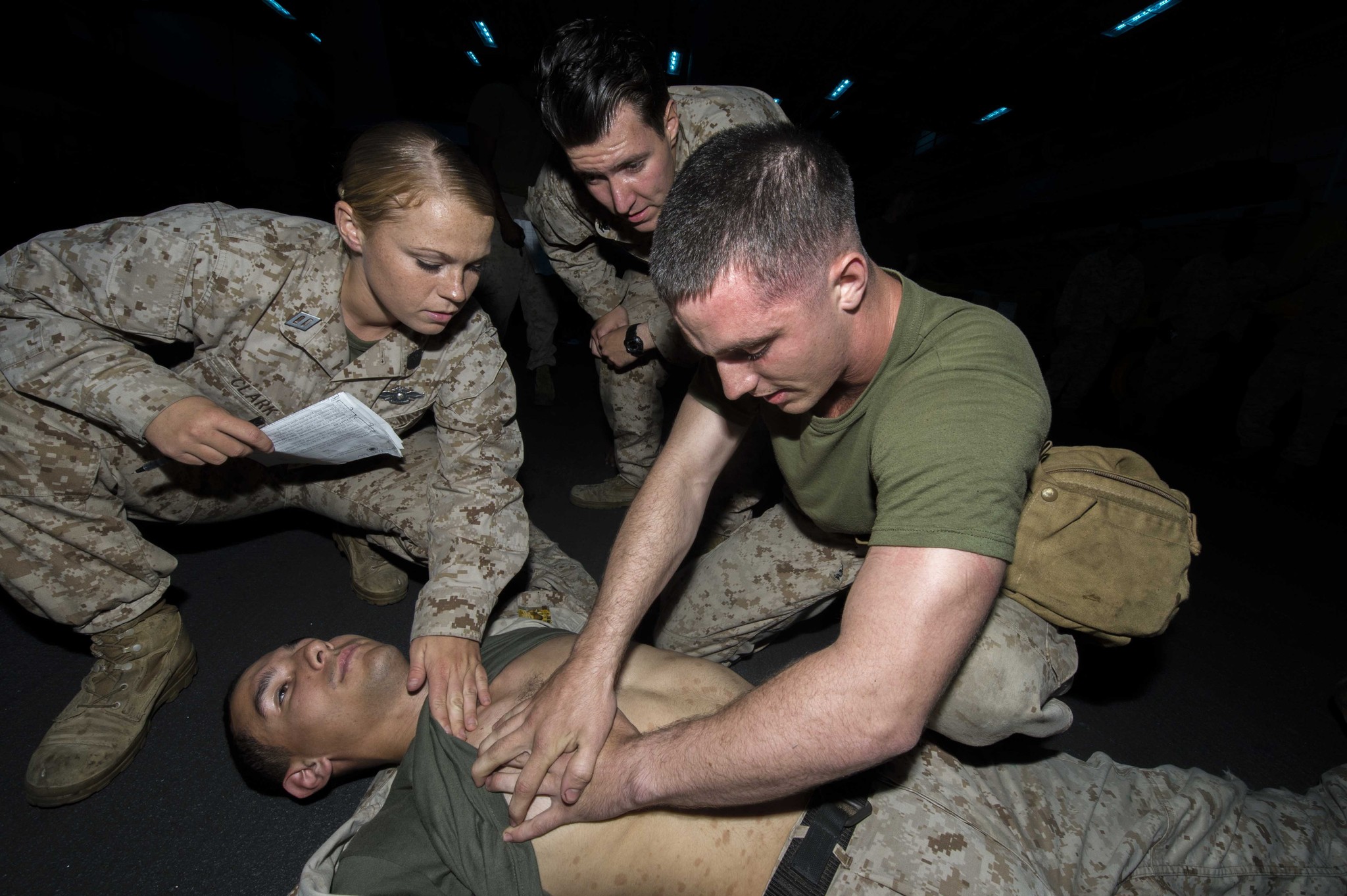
[1008,440,1198,644]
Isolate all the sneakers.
[570,475,640,509]
[337,526,411,605]
[25,603,199,805]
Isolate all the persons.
[473,125,1201,843]
[225,620,1346,895]
[0,120,530,808]
[523,13,794,565]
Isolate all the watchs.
[623,322,647,360]
